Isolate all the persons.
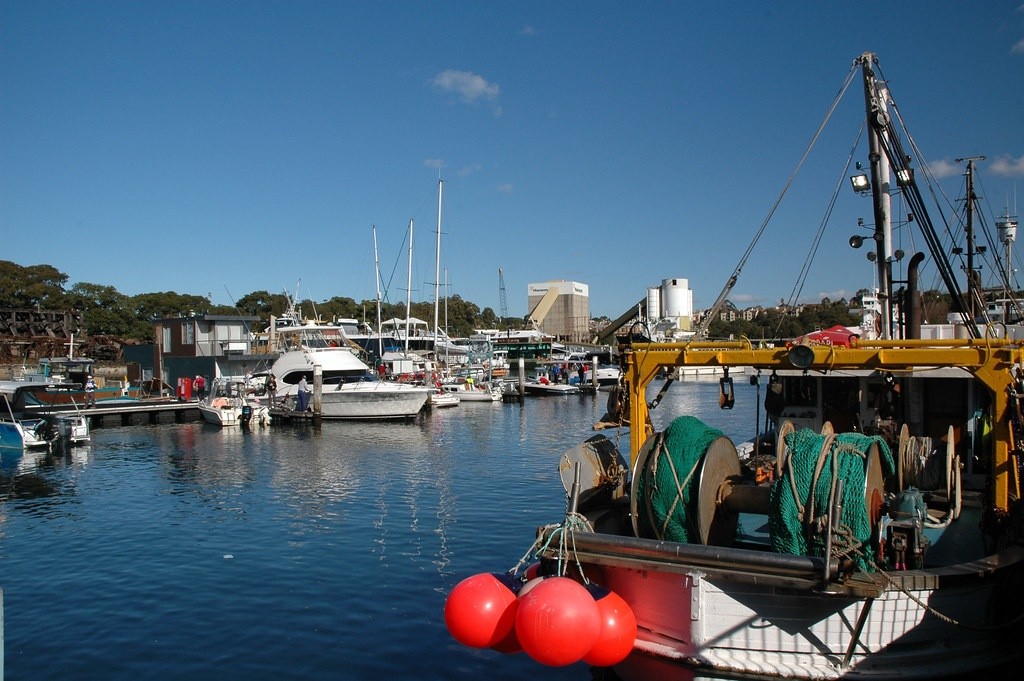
[34,419,53,441]
[83,375,98,410]
[280,392,295,413]
[328,338,392,381]
[266,377,278,409]
[297,375,312,413]
[192,371,251,412]
[551,362,587,385]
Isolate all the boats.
[0,176,626,481]
[501,52,1022,681]
